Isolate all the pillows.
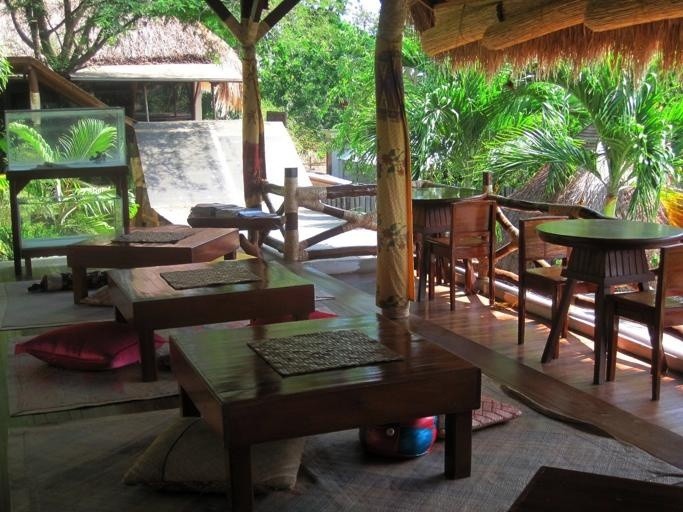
[15,321,171,371]
[119,416,306,493]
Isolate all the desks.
[167,313,481,512]
[535,220,682,385]
[5,164,130,281]
[409,189,488,302]
[64,226,314,383]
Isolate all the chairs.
[606,240,683,399]
[516,213,601,362]
[411,180,431,187]
[426,198,501,313]
